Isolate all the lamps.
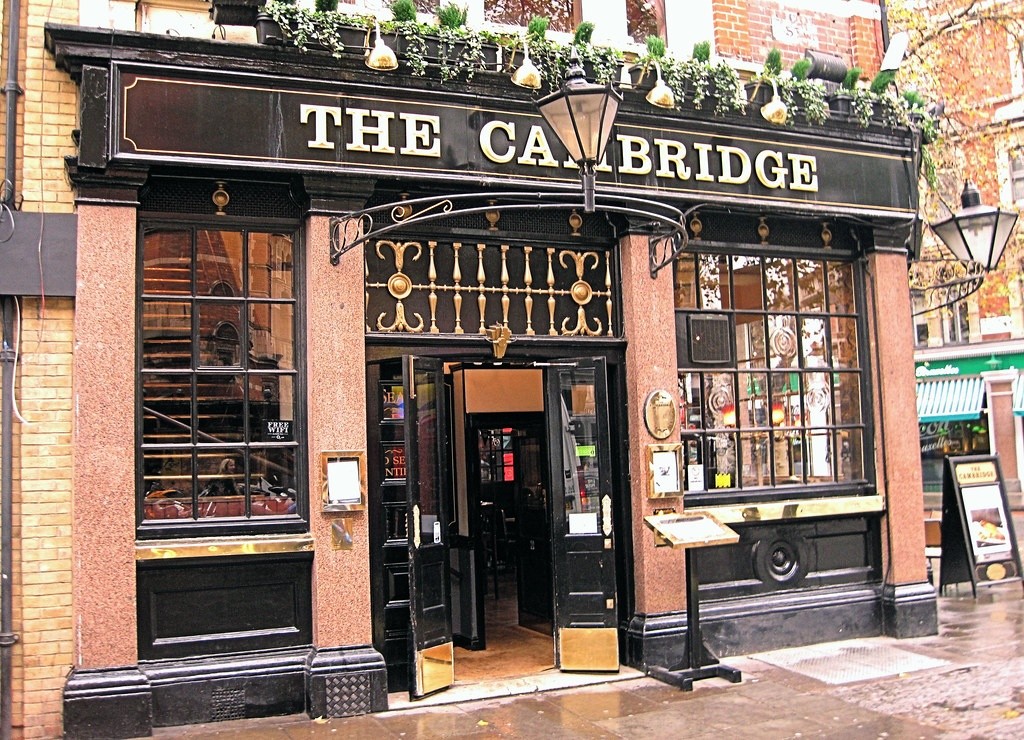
[760,77,788,123]
[639,59,676,108]
[534,47,625,212]
[208,0,266,26]
[511,44,542,90]
[804,50,847,82]
[913,178,1019,319]
[365,20,398,71]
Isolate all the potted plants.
[254,0,915,125]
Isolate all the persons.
[208,458,240,495]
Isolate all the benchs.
[925,518,959,591]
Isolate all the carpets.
[451,625,555,680]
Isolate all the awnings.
[1013,373,1024,416]
[918,377,984,424]
